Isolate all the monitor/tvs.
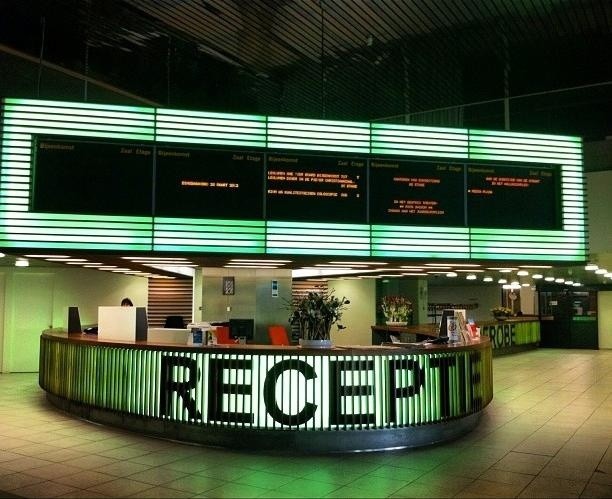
[30,135,154,223]
[466,162,563,235]
[146,327,191,346]
[153,139,264,223]
[265,149,368,227]
[367,154,467,233]
[229,319,254,344]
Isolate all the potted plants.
[277,288,350,350]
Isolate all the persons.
[121,298,133,307]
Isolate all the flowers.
[381,295,413,322]
[490,305,511,316]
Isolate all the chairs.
[216,326,240,344]
[268,326,290,345]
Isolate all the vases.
[496,316,508,321]
[386,321,408,327]
